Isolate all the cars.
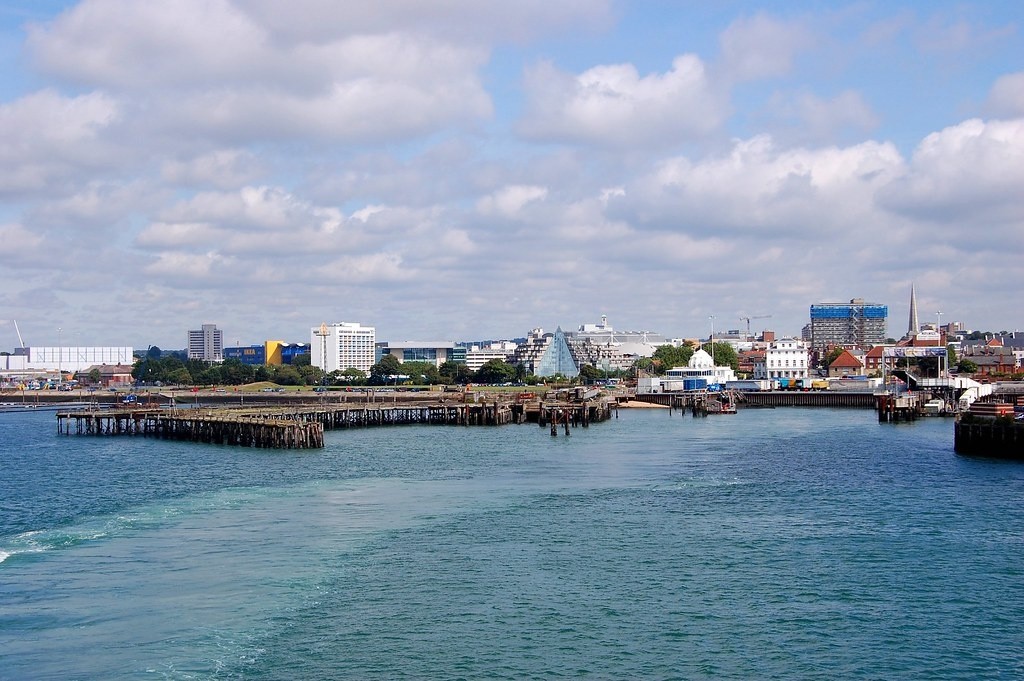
[58,380,117,392]
[262,387,286,392]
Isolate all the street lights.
[935,309,943,347]
[708,315,716,357]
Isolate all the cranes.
[739,313,771,330]
